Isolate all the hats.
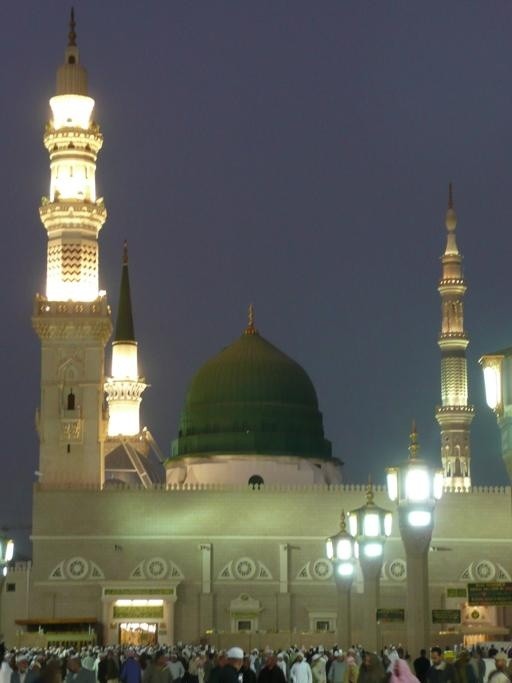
[249,644,362,661]
[493,653,508,662]
[226,646,244,661]
[4,646,106,671]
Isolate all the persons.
[0,639,512,683]
